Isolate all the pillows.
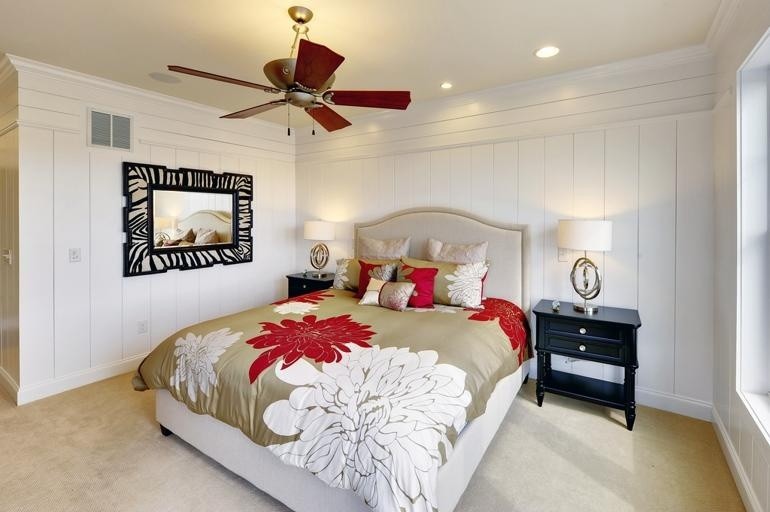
[333,235,491,312]
[154,226,219,246]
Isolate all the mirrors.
[122,161,254,277]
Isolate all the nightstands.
[532,298,642,431]
[286,271,336,299]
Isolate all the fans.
[166,7,411,133]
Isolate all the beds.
[132,207,531,511]
[153,211,233,249]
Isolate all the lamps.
[555,219,613,314]
[304,221,335,277]
[285,90,316,108]
